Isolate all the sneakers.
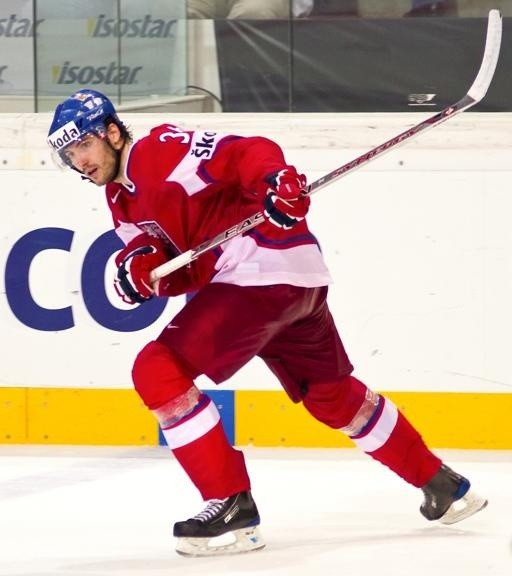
[171,487,262,539]
[419,463,471,521]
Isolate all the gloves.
[115,231,170,307]
[261,165,311,230]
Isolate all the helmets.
[46,89,117,173]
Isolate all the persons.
[44,88,471,538]
[185,1,315,20]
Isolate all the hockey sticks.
[149,7,503,284]
